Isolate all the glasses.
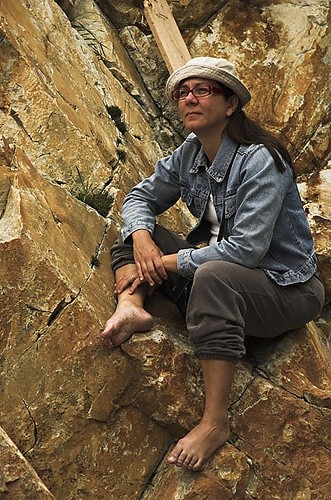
[171,83,232,99]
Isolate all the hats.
[165,57,252,109]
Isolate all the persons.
[102,56,325,473]
